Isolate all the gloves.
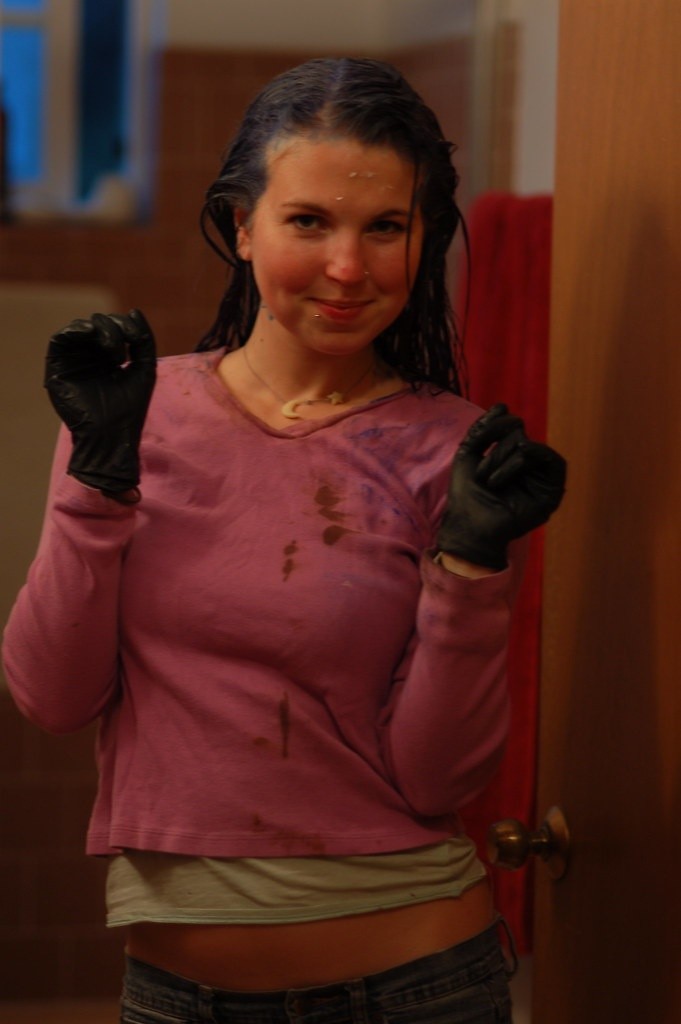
[45,309,157,506]
[435,403,567,572]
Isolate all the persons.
[0,56,566,1024]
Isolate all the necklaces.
[243,346,371,418]
